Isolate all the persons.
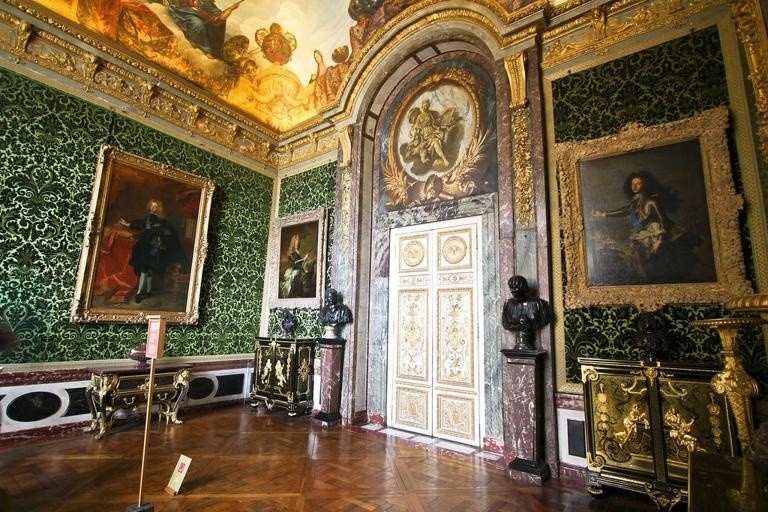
[590,170,688,284]
[664,406,703,452]
[499,276,551,331]
[118,198,189,303]
[317,288,352,326]
[404,99,449,166]
[279,233,310,298]
[161,0,350,114]
[612,403,650,449]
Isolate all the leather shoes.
[135,291,152,303]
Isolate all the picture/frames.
[266,206,328,310]
[552,104,753,315]
[71,142,218,328]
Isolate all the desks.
[84,361,193,440]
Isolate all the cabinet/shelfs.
[251,337,315,416]
[576,357,739,511]
